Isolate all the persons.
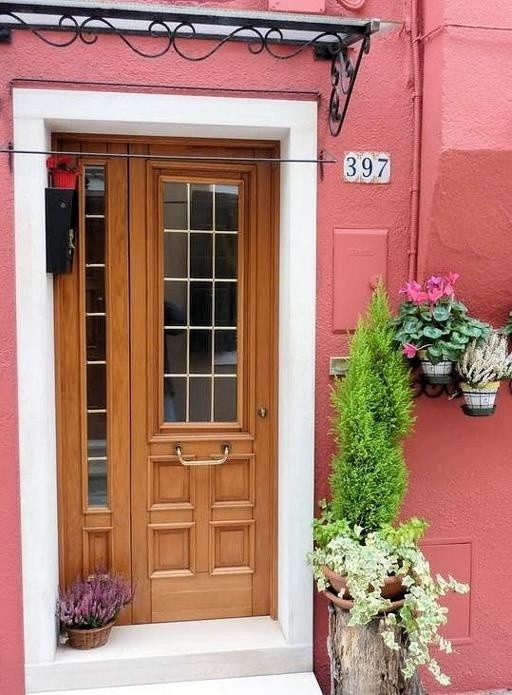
[163,298,185,423]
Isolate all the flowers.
[392,271,489,365]
[57,566,136,629]
[456,327,512,390]
[46,154,81,175]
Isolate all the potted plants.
[309,496,473,688]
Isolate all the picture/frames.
[85,286,99,349]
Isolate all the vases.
[416,350,454,384]
[62,618,117,651]
[457,381,500,416]
[52,168,76,189]
